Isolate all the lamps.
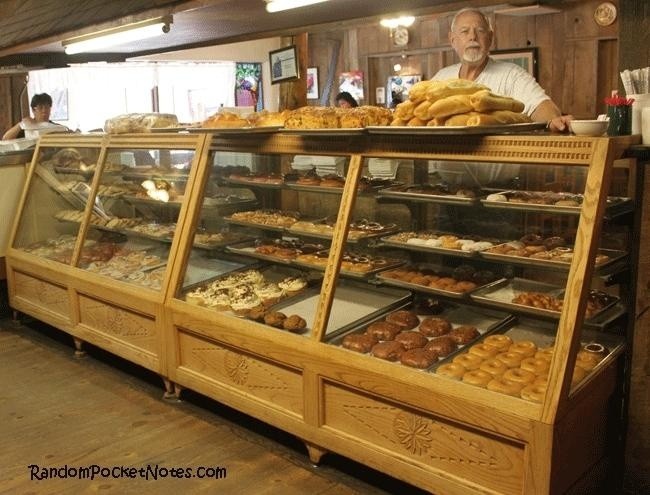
[61,15,173,54]
[379,14,416,29]
[265,0,331,14]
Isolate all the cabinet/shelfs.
[5,135,206,399]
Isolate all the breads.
[426,119,445,126]
[445,114,470,126]
[385,311,420,329]
[425,337,459,357]
[392,118,406,126]
[395,332,428,351]
[366,323,401,340]
[193,233,258,248]
[408,80,449,103]
[382,259,501,293]
[493,233,603,264]
[373,341,407,361]
[264,313,286,327]
[22,234,98,255]
[449,326,481,346]
[245,244,306,263]
[285,105,392,129]
[184,268,317,315]
[190,162,250,180]
[248,111,284,129]
[402,349,439,368]
[419,318,452,337]
[355,176,372,191]
[297,166,320,188]
[436,335,602,404]
[53,208,143,231]
[413,101,434,120]
[395,100,417,120]
[470,90,525,113]
[291,221,402,240]
[104,112,177,133]
[129,268,167,289]
[429,95,470,119]
[389,180,487,200]
[85,251,162,278]
[512,290,619,318]
[200,111,251,130]
[342,334,378,353]
[50,148,117,174]
[382,233,514,252]
[298,249,406,275]
[426,80,490,101]
[137,162,189,177]
[227,172,284,187]
[50,241,122,267]
[135,180,255,204]
[283,315,307,332]
[407,117,425,127]
[321,173,344,187]
[484,191,623,210]
[229,210,298,229]
[467,111,533,127]
[248,306,268,321]
[131,223,205,239]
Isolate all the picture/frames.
[166,132,642,495]
[487,46,538,81]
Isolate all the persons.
[430,8,576,132]
[2,94,60,139]
[337,91,357,108]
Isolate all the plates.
[88,123,185,133]
[89,207,157,235]
[480,189,632,215]
[189,122,283,134]
[121,169,192,182]
[326,292,514,373]
[283,176,406,195]
[469,272,620,321]
[377,182,508,205]
[379,226,514,259]
[121,213,278,250]
[295,243,410,276]
[224,230,330,266]
[119,252,260,293]
[124,222,207,239]
[258,278,413,345]
[179,261,330,318]
[112,254,261,298]
[375,260,510,297]
[223,174,285,188]
[56,166,124,173]
[158,225,270,250]
[366,123,547,134]
[478,243,629,268]
[279,128,365,136]
[77,244,208,280]
[285,217,407,244]
[221,209,331,231]
[426,314,628,406]
[56,165,127,176]
[125,195,261,219]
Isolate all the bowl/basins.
[570,120,610,137]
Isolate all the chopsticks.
[620,67,649,94]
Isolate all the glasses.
[35,106,50,110]
